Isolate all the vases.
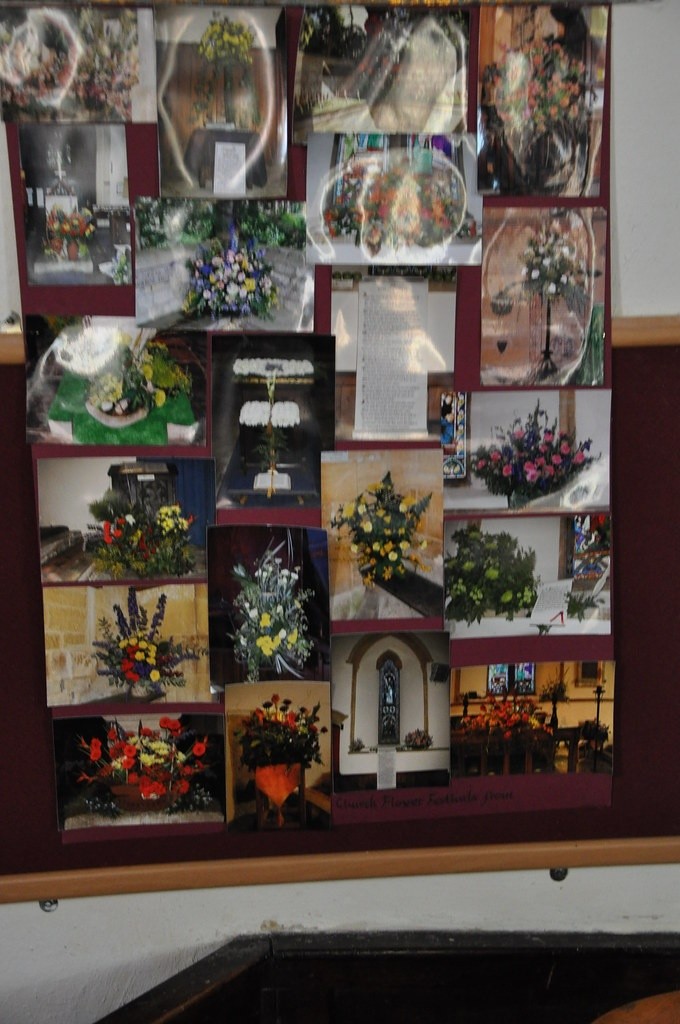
[85,402,150,429]
[110,785,175,812]
[132,682,151,697]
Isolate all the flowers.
[484,37,593,141]
[176,224,280,319]
[470,398,603,509]
[188,12,263,122]
[1,7,142,121]
[326,146,477,251]
[41,206,99,261]
[86,488,200,578]
[444,520,542,626]
[90,343,197,418]
[226,534,318,684]
[232,693,329,775]
[491,222,604,314]
[329,471,435,593]
[460,690,554,743]
[76,716,208,808]
[404,728,432,750]
[89,585,209,697]
